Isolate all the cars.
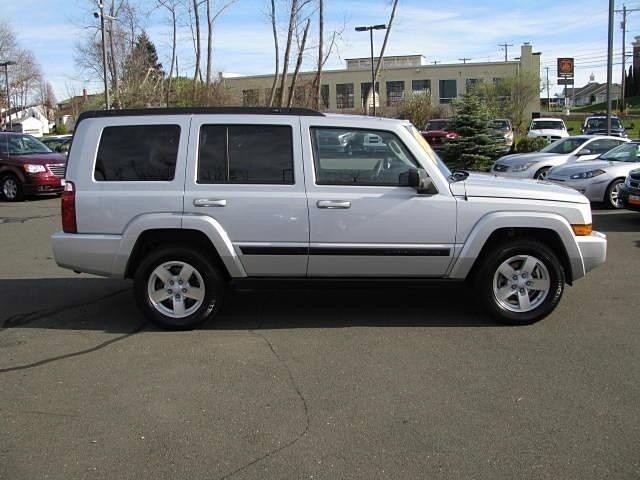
[40,134,73,157]
[360,132,391,154]
[618,168,639,212]
[316,130,360,156]
[0,132,67,201]
[483,112,640,209]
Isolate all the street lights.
[355,25,388,117]
[0,61,16,132]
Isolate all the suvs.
[51,107,608,330]
[419,118,460,158]
